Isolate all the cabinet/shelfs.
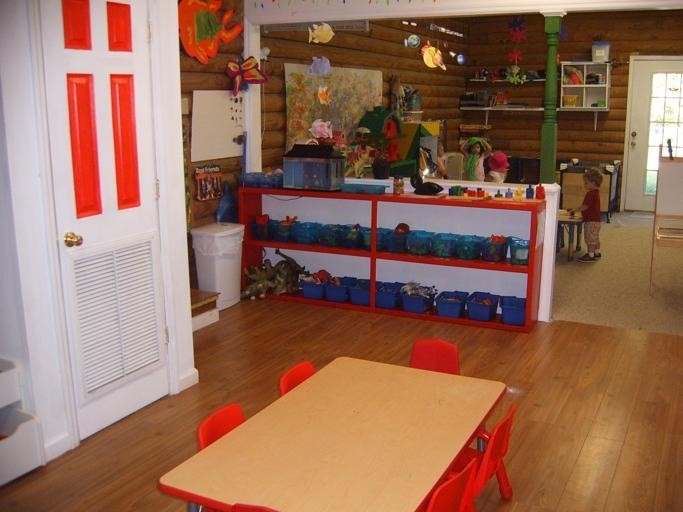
[559,60,610,112]
[238,185,546,334]
[459,77,560,111]
[560,170,619,223]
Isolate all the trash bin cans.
[190,222,245,312]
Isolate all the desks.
[558,209,584,261]
[157,358,507,512]
[509,157,572,184]
[360,158,417,178]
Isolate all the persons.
[482,151,511,183]
[565,168,603,263]
[459,136,492,183]
[437,140,447,180]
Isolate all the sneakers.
[575,253,601,263]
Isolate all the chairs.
[187,401,246,512]
[417,400,516,511]
[278,359,317,399]
[408,339,461,378]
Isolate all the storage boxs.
[592,41,610,62]
[247,219,530,327]
[402,111,424,122]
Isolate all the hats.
[465,137,485,149]
[487,151,510,173]
